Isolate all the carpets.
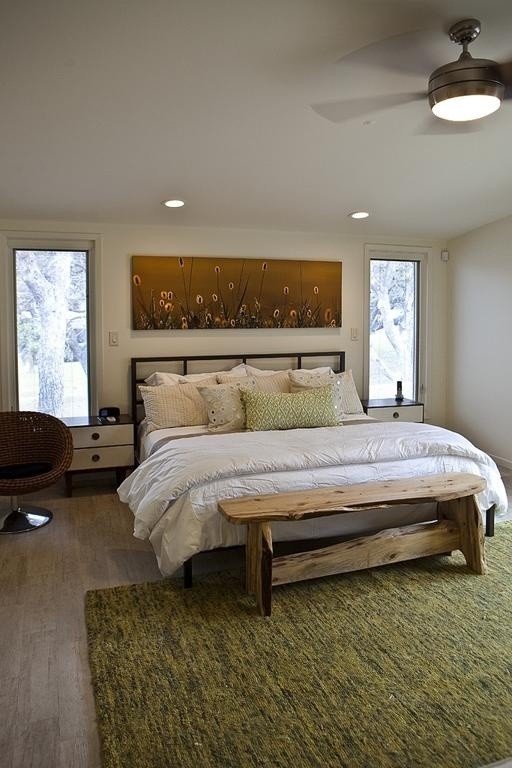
[85,521,512,768]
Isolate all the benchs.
[218,473,487,617]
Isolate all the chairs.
[0,411,73,535]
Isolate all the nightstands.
[58,414,134,497]
[361,397,424,423]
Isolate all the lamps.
[427,58,504,123]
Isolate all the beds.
[115,351,509,588]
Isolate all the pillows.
[196,377,256,433]
[243,364,334,376]
[288,370,365,416]
[144,363,245,386]
[239,384,342,432]
[138,374,218,436]
[216,371,290,393]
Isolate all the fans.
[310,18,512,135]
